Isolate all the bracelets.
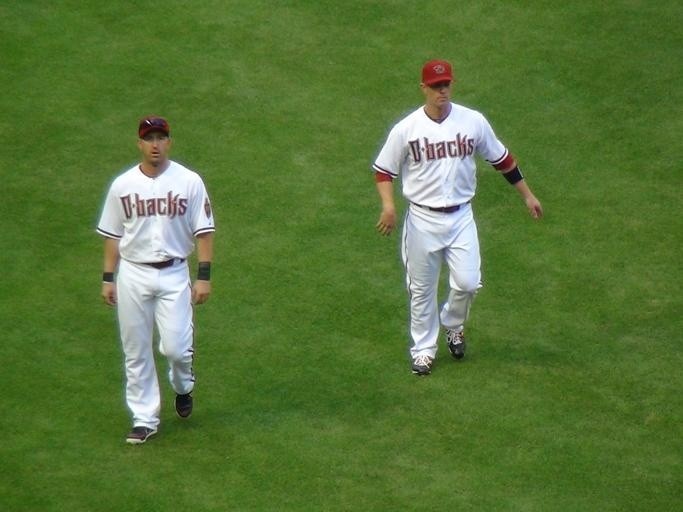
[102,272,114,283]
[197,261,210,281]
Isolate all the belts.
[414,200,470,213]
[142,258,185,269]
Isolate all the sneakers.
[446,327,465,359]
[175,391,193,418]
[126,424,157,444]
[411,356,433,375]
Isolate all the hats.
[422,60,452,86]
[139,116,169,137]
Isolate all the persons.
[373,59,543,376]
[96,114,216,444]
